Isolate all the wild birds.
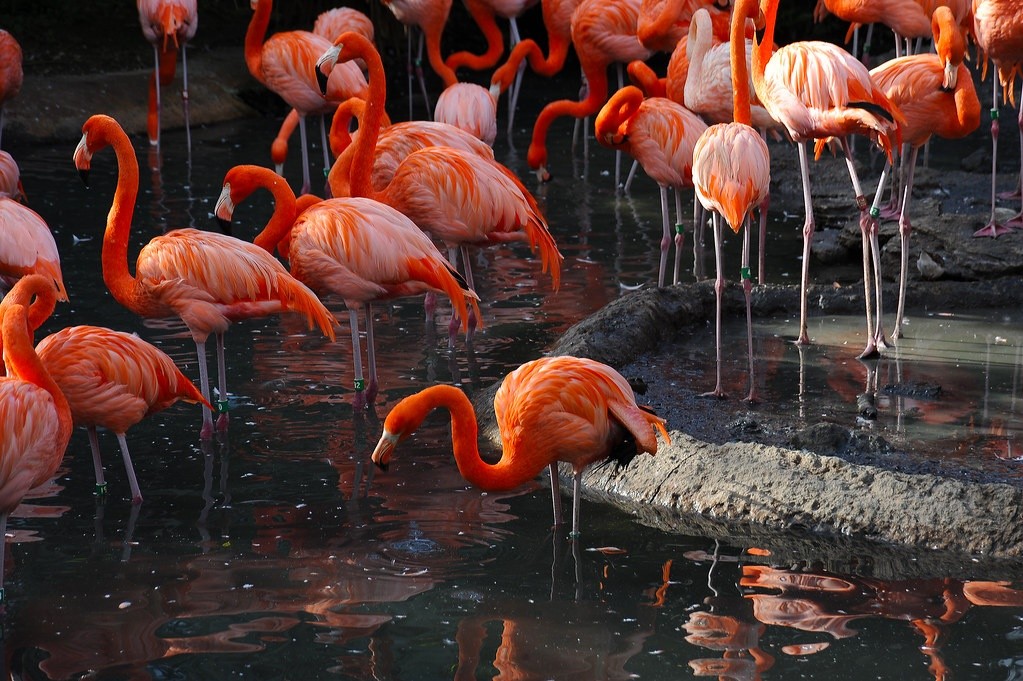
[0,0,1022,545]
